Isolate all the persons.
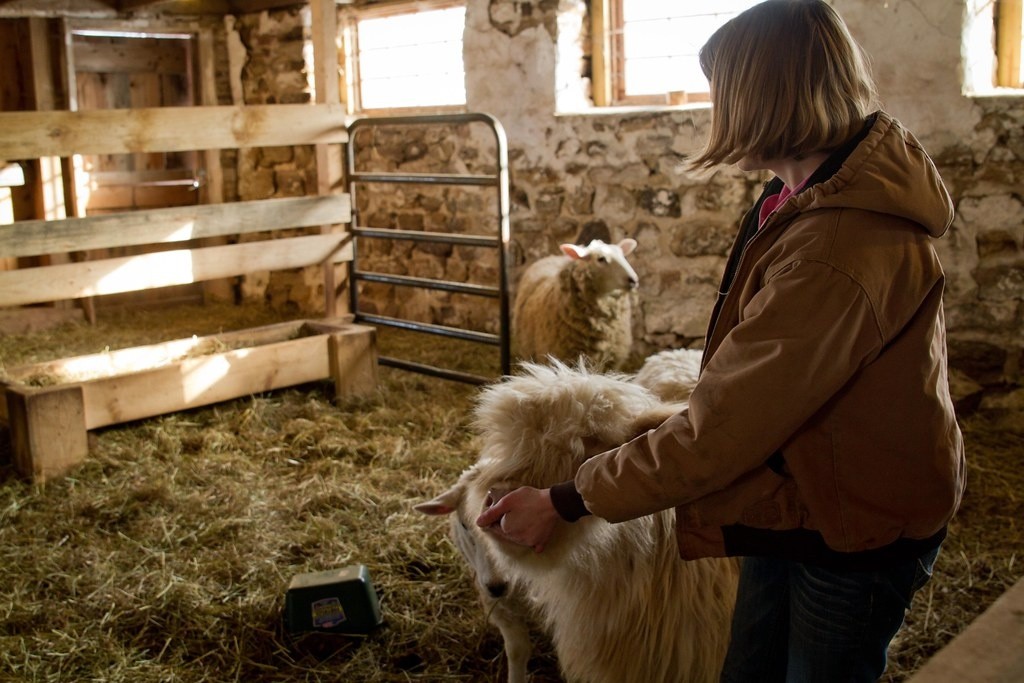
[476,0,966,683]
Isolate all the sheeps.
[507,236,640,378]
[409,345,742,683]
